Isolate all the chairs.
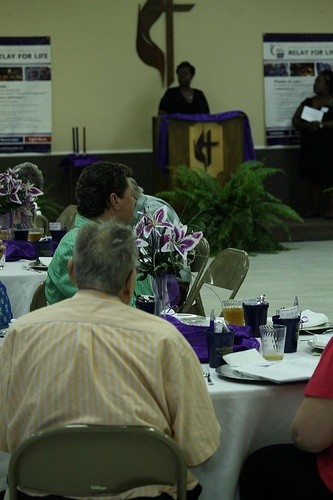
[176,236,249,315]
[6,422,187,500]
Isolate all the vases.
[151,272,170,318]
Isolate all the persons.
[288,337,333,500]
[0,240,13,328]
[130,178,192,284]
[46,161,153,307]
[0,223,221,500]
[292,69,333,218]
[158,62,210,115]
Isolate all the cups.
[13,229,29,242]
[28,228,44,241]
[222,299,244,326]
[204,326,236,368]
[50,230,65,242]
[242,302,269,338]
[259,324,286,361]
[272,315,300,353]
[135,296,155,315]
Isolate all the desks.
[187,313,333,500]
[0,257,52,331]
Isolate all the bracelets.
[319,123,323,128]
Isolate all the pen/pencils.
[294,296,298,306]
[257,294,268,305]
[210,309,214,332]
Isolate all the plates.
[308,340,325,350]
[215,363,268,380]
[300,322,330,330]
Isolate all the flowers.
[131,206,205,274]
[0,168,43,218]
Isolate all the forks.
[203,367,214,385]
